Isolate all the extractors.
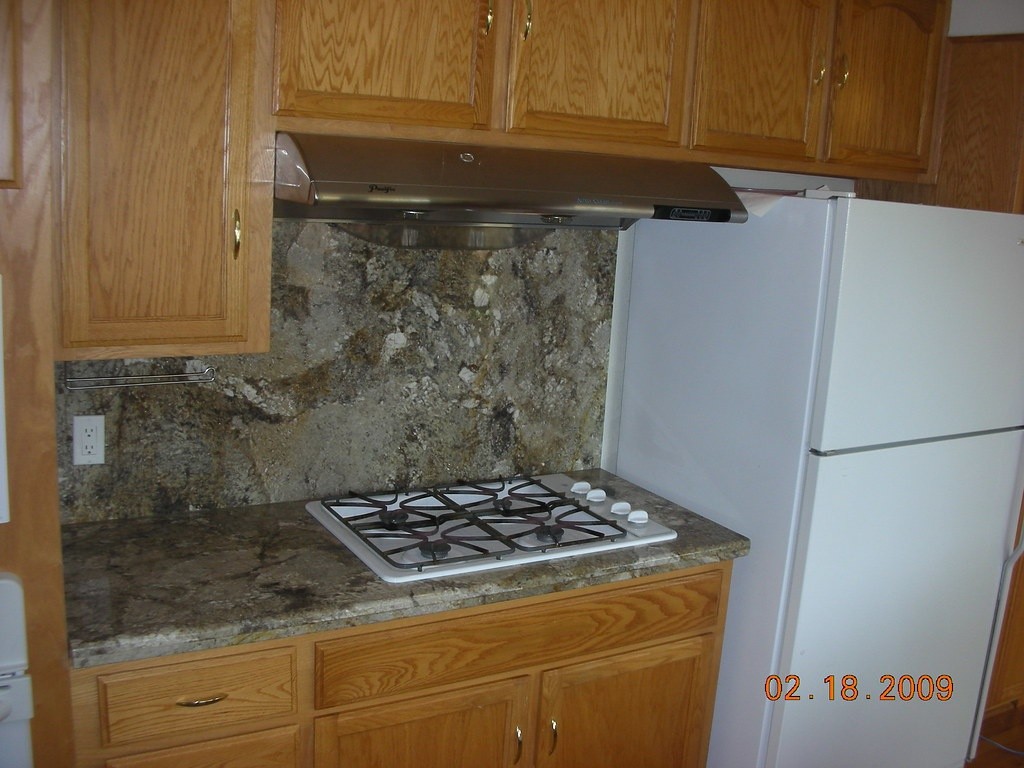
[272,130,749,251]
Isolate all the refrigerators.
[598,165,1024,768]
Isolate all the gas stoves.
[304,472,678,584]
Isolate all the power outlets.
[71,415,104,465]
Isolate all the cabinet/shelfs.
[68,632,314,768]
[690,0,945,173]
[314,558,733,768]
[54,0,276,361]
[270,1,693,147]
